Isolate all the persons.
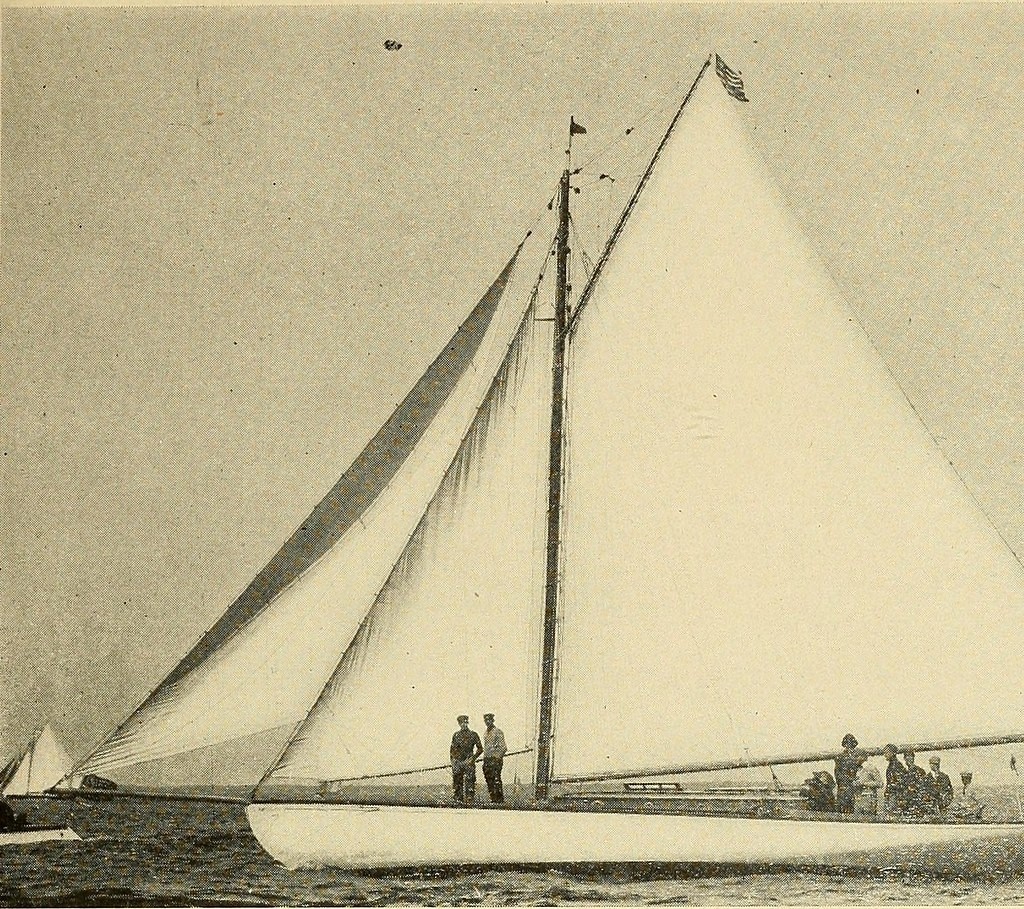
[483,714,507,803]
[799,733,984,819]
[450,714,483,804]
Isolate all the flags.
[716,53,749,102]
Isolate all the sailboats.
[0,55,1024,875]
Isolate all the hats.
[929,756,940,764]
[960,770,972,778]
[841,734,857,749]
[484,713,495,720]
[457,715,468,723]
